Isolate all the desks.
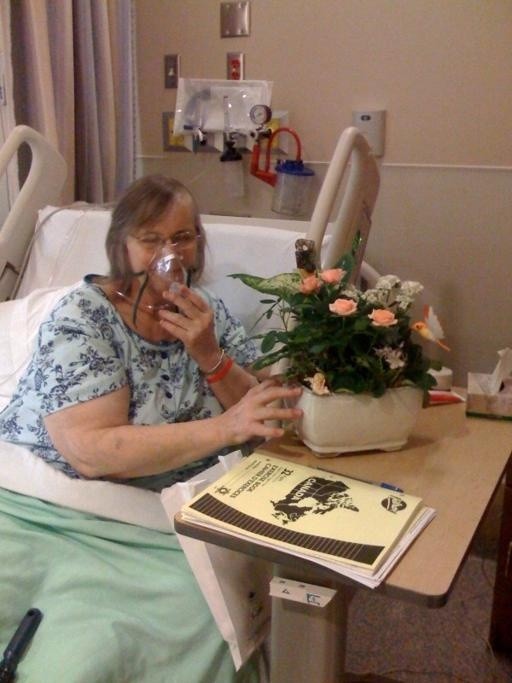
[174,385,512,683]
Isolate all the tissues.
[466,348,512,420]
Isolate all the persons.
[0,171,303,494]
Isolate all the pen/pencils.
[318,466,404,492]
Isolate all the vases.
[282,371,426,457]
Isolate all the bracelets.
[198,344,226,376]
[205,356,234,384]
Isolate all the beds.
[0,124,399,683]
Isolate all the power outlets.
[227,51,245,81]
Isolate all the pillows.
[0,275,87,408]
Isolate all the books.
[183,451,425,577]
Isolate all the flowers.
[225,265,450,408]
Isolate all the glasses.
[129,230,201,249]
